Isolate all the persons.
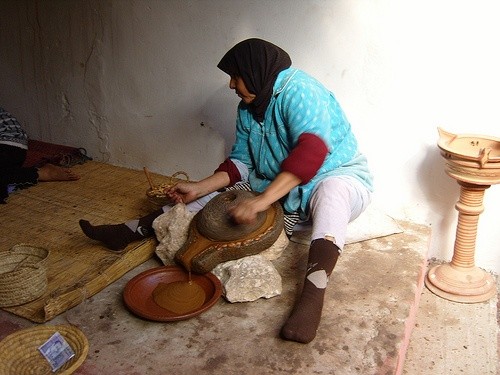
[0,105,80,184]
[79,38,373,344]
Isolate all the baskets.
[0,325,88,375]
[0,244,51,308]
[145,170,189,212]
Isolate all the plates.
[123,266,223,322]
